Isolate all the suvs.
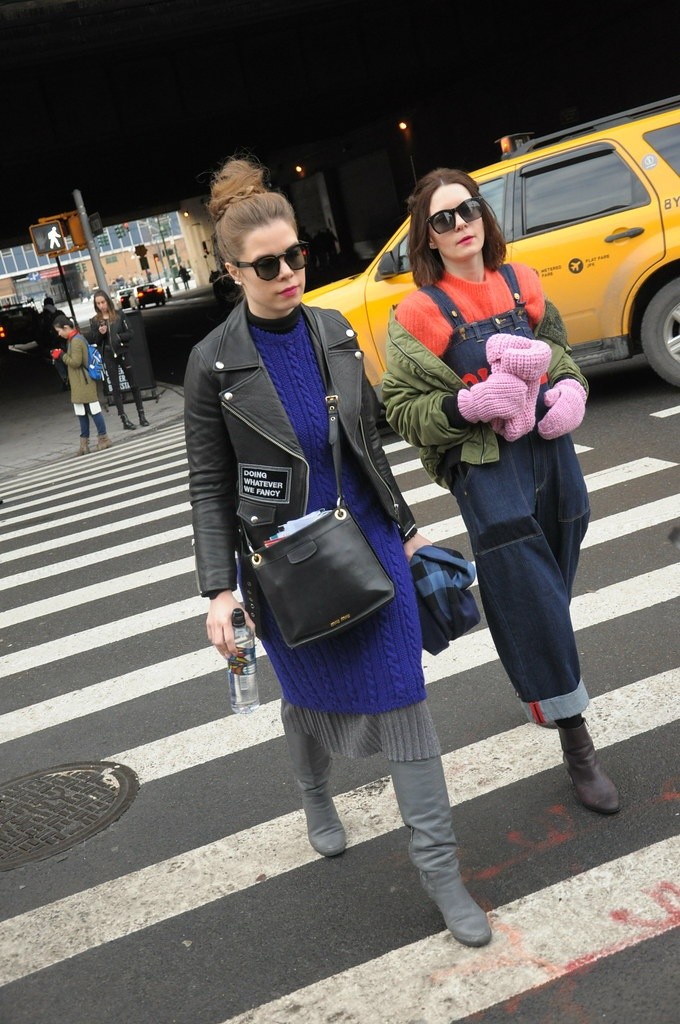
[301,92,680,434]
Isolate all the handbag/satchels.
[251,495,396,648]
[186,273,190,280]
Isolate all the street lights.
[399,121,417,187]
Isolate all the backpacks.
[72,334,103,383]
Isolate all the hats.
[486,334,551,441]
[44,298,54,306]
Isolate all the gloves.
[537,379,588,440]
[50,348,63,360]
[458,373,528,423]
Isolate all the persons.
[298,224,339,269]
[40,296,80,391]
[184,152,496,949]
[89,290,150,431]
[129,293,138,310]
[49,315,112,457]
[177,266,191,289]
[381,167,621,817]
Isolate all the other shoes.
[62,377,71,392]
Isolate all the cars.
[135,284,166,309]
[110,288,134,311]
[0,301,48,361]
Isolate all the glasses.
[234,240,310,280]
[426,197,484,234]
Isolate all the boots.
[544,722,557,728]
[554,717,622,813]
[139,410,149,426]
[75,436,90,456]
[93,433,112,452]
[389,756,491,948]
[283,726,347,856]
[120,414,137,430]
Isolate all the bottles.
[226,608,260,714]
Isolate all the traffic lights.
[28,220,66,256]
[153,254,160,261]
